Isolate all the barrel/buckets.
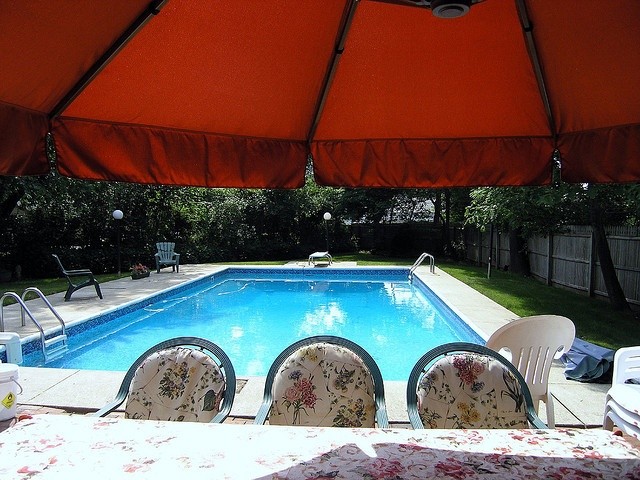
[0,363,21,423]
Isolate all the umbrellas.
[0,2,639,189]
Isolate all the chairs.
[154,242,180,273]
[90,336,237,423]
[485,315,576,429]
[602,345,640,441]
[406,342,549,429]
[251,335,390,428]
[52,254,103,302]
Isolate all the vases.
[133,271,149,278]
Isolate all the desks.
[308,251,333,266]
[0,413,640,480]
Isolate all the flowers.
[129,263,147,274]
